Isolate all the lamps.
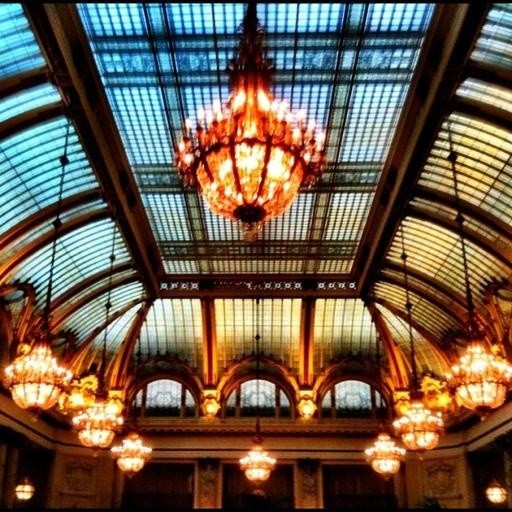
[173,4,325,244]
[2,108,72,413]
[62,111,125,460]
[109,117,152,479]
[441,110,511,420]
[388,203,449,455]
[362,292,408,479]
[237,291,278,488]
